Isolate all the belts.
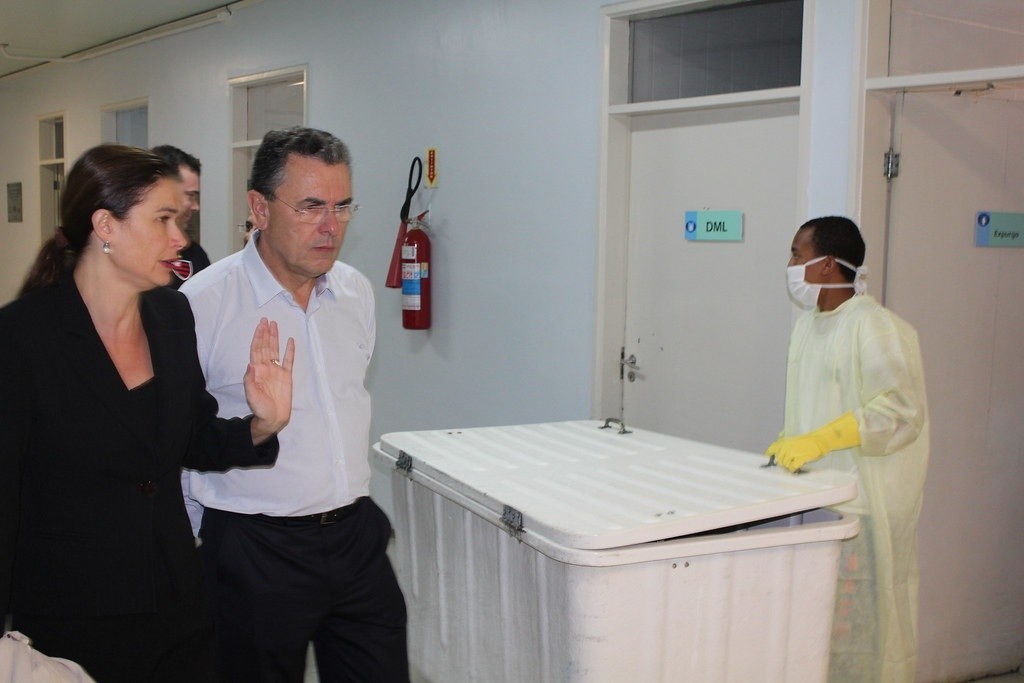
[249,498,352,524]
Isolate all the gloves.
[764,413,864,472]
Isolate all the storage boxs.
[372,417,861,683]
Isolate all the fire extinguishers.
[385,155,433,330]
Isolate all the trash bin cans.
[369,415,863,683]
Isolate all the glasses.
[270,191,362,222]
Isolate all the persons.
[760,216,931,683]
[149,146,211,288]
[0,143,295,683]
[178,126,409,682]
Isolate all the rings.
[270,359,279,364]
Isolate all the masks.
[785,255,829,312]
